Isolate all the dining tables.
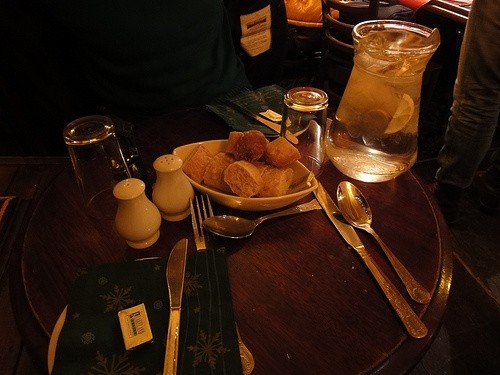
[8,107,453,375]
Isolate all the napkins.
[204,84,317,137]
[52,248,244,375]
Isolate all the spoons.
[203,198,321,238]
[336,181,432,304]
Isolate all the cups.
[63,115,132,218]
[281,86,329,179]
[326,18,441,184]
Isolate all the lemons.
[384,94,415,134]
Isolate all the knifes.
[162,239,189,375]
[312,177,428,338]
[214,92,299,145]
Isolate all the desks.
[396,0,472,23]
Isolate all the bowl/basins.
[172,138,319,212]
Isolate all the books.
[206,84,316,138]
[50,248,242,375]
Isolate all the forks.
[187,195,255,375]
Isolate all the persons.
[431,0,500,207]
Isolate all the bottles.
[146,147,194,224]
[111,177,162,250]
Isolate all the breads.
[182,131,301,198]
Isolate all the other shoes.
[429,182,460,224]
[484,186,500,214]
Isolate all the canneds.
[280,87,329,178]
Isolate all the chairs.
[322,0,379,59]
